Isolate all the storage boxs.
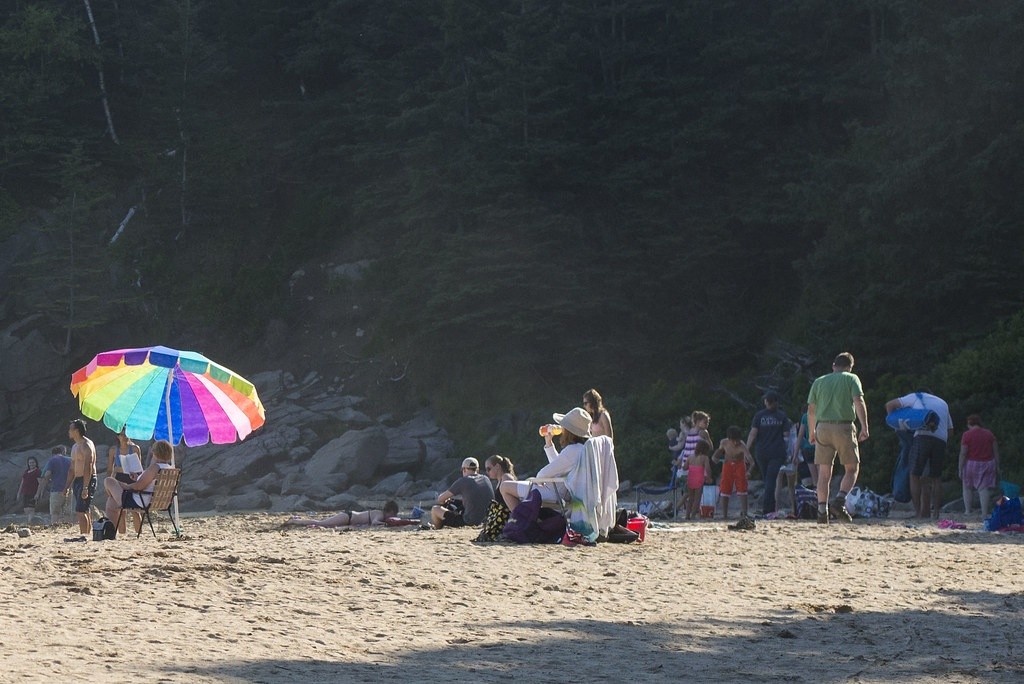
[627,519,646,542]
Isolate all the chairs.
[633,462,681,519]
[524,437,615,528]
[114,468,181,538]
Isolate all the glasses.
[486,465,496,472]
[583,402,591,405]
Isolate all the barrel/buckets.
[627,519,646,542]
[702,506,714,518]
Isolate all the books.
[119,453,143,473]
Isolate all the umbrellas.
[70,345,265,536]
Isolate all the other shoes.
[832,495,852,524]
[817,514,829,525]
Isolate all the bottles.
[538,424,562,437]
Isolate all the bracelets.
[83,486,88,490]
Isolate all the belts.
[818,420,852,424]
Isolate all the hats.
[553,407,593,438]
[761,390,781,401]
[462,457,479,469]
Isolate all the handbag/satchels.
[984,496,1023,531]
[502,487,566,545]
[794,481,895,519]
[93,516,116,541]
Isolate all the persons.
[886,387,953,520]
[958,414,1000,519]
[581,388,612,439]
[34,444,71,524]
[672,411,713,520]
[16,456,42,515]
[282,500,398,526]
[745,390,790,517]
[710,426,755,519]
[431,457,495,529]
[499,407,593,511]
[472,455,517,541]
[667,415,693,513]
[104,440,174,538]
[62,419,97,534]
[807,352,869,524]
[107,424,143,536]
[793,406,820,488]
